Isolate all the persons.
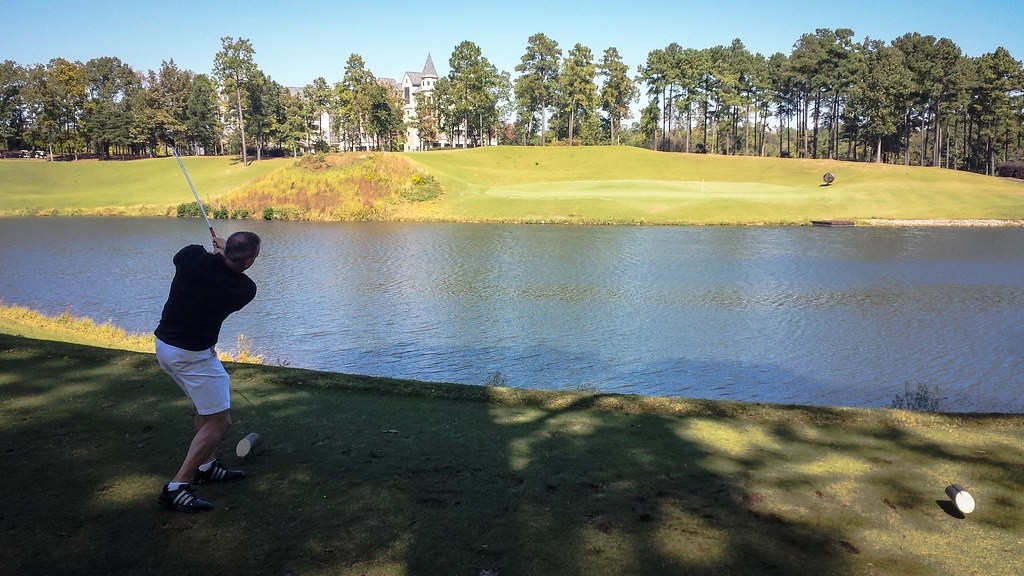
[153,231,261,512]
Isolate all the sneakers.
[197,459,245,484]
[158,480,214,513]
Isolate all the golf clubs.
[162,134,218,249]
